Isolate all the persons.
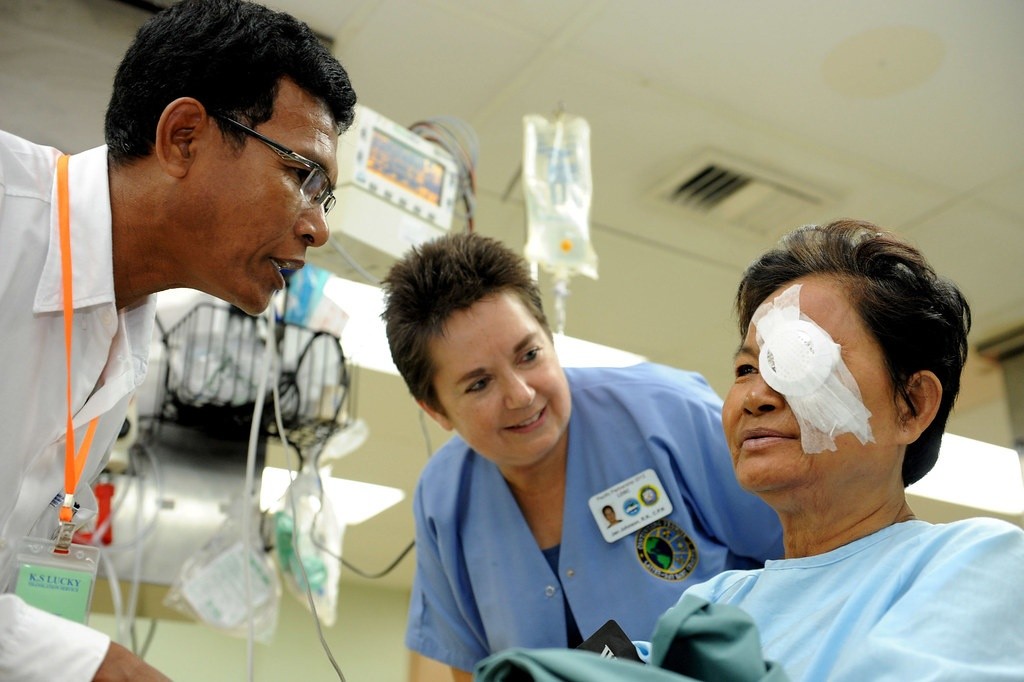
[382,229,784,682]
[602,505,622,528]
[630,220,1024,682]
[1,1,358,681]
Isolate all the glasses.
[212,113,336,217]
